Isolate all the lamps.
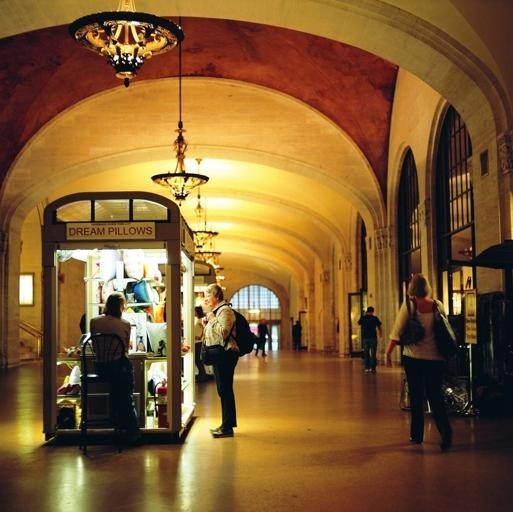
[68,0,184,88]
[150,16,226,293]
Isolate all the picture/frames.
[18,271,35,306]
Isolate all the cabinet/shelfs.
[84,254,188,428]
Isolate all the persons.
[357,306,382,374]
[90,294,142,435]
[292,320,298,352]
[194,282,232,437]
[255,319,271,357]
[384,274,454,453]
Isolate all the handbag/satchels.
[431,300,458,359]
[200,343,212,365]
[395,295,425,346]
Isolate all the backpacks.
[227,306,254,354]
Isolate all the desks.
[82,348,147,428]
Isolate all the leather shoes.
[210,427,220,433]
[213,429,234,437]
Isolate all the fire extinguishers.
[153,378,167,428]
